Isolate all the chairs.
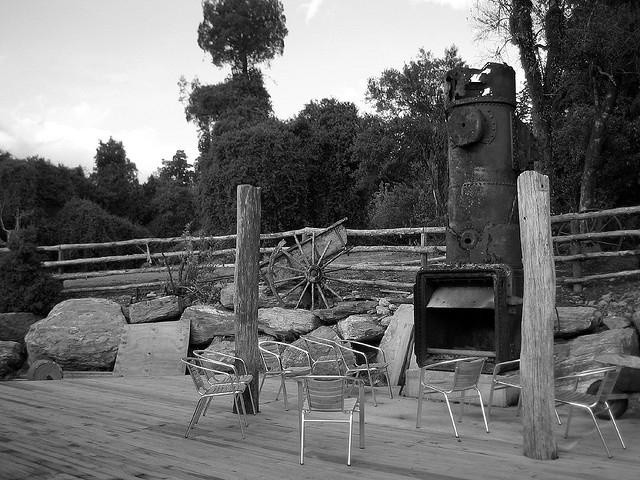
[412,356,627,460]
[181,335,394,465]
